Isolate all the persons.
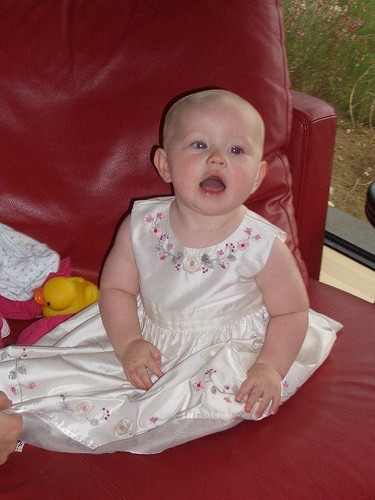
[66,88,310,434]
[0,390,23,466]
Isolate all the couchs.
[0,0,375,500]
